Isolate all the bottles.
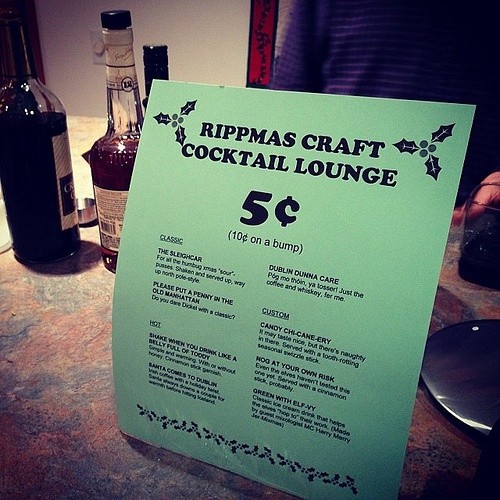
[89,10,144,273]
[142,44,169,112]
[0,8,81,264]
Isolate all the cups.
[458,182,500,292]
